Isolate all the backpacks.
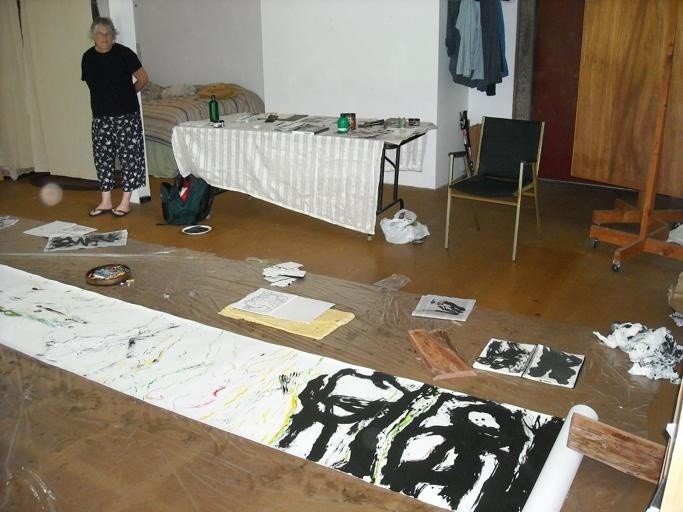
[160,173,214,226]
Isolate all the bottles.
[208,94,219,122]
[336,113,350,133]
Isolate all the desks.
[175,112,436,241]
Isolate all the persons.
[77,18,150,219]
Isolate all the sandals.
[89,208,112,217]
[111,207,131,217]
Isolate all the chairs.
[444,115,547,263]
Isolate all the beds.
[141,83,258,181]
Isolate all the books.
[357,116,438,135]
[291,123,329,137]
